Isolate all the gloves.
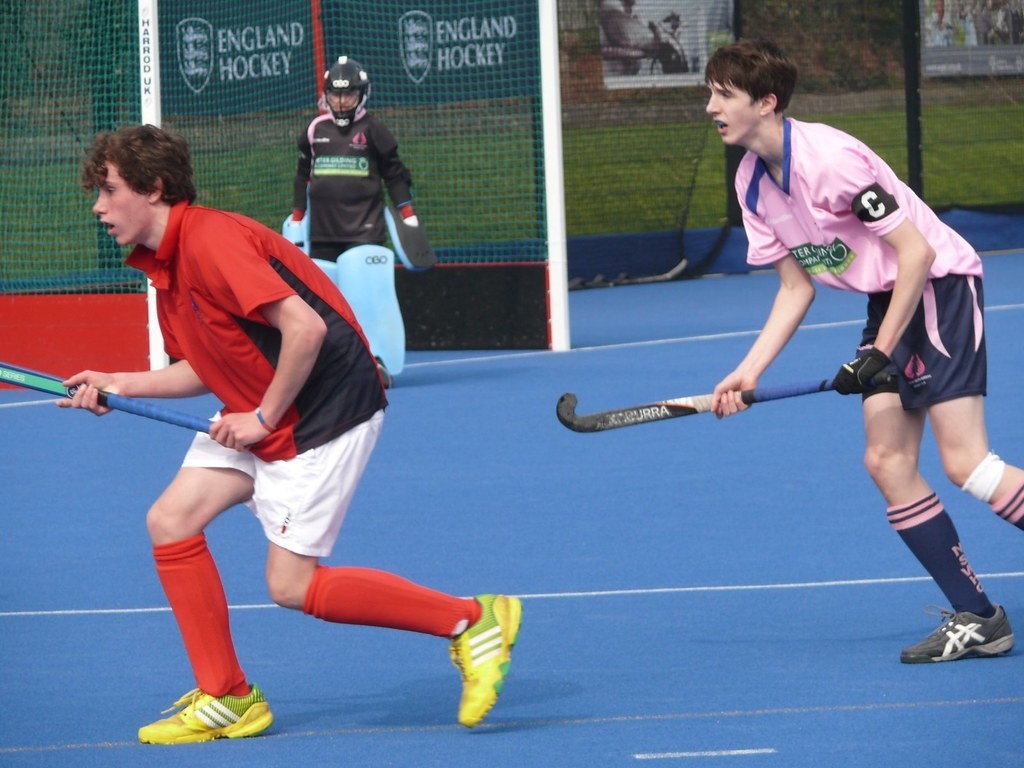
[832,344,895,396]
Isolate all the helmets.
[323,55,371,132]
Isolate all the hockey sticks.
[0,361,216,434]
[555,370,893,434]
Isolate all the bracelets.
[255,408,277,434]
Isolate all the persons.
[292,55,439,273]
[58,126,521,746]
[703,40,1024,667]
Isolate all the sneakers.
[138,682,274,745]
[450,593,521,728]
[901,604,1016,664]
